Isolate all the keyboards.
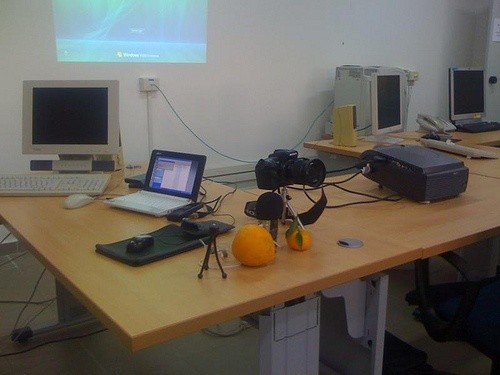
[463,121,500,133]
[0,176,109,197]
[421,138,499,159]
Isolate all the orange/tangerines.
[231,223,275,266]
[287,230,312,251]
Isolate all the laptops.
[104,150,208,217]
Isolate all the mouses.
[422,133,440,141]
[126,235,154,253]
[65,194,95,209]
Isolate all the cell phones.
[168,203,203,222]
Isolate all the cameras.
[256,149,326,189]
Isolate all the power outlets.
[140,77,160,91]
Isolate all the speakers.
[333,105,357,148]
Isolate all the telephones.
[416,113,456,133]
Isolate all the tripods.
[198,224,227,279]
[280,185,305,230]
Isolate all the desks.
[0,130,500,375]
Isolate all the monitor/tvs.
[447,66,486,125]
[335,65,406,135]
[21,80,118,174]
